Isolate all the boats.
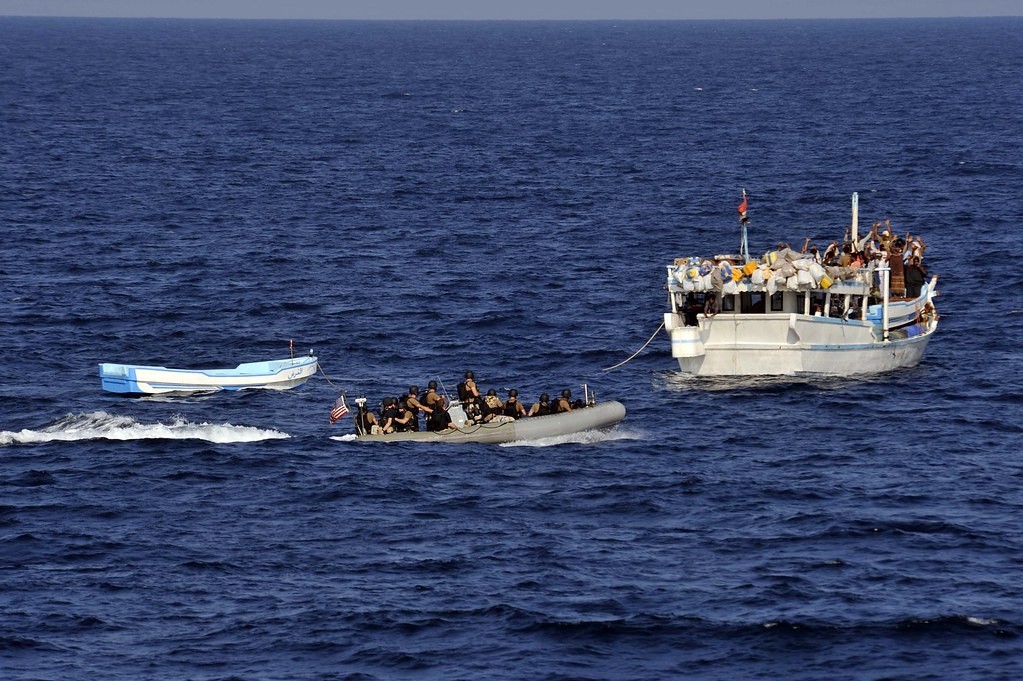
[663,190,940,381]
[99,339,318,398]
[350,400,627,447]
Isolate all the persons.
[377,397,396,435]
[391,398,415,432]
[426,381,445,406]
[406,386,434,429]
[485,389,506,414]
[504,389,527,420]
[558,389,573,412]
[777,219,928,319]
[684,292,720,325]
[355,405,378,434]
[465,372,489,414]
[528,394,550,416]
[429,398,456,431]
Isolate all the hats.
[882,231,889,237]
[912,242,921,248]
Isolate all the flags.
[331,395,348,423]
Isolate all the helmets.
[410,386,418,394]
[509,388,517,396]
[540,393,549,402]
[465,371,474,379]
[561,388,571,398]
[488,389,496,396]
[358,404,366,410]
[391,397,398,405]
[428,381,437,388]
[383,397,393,406]
